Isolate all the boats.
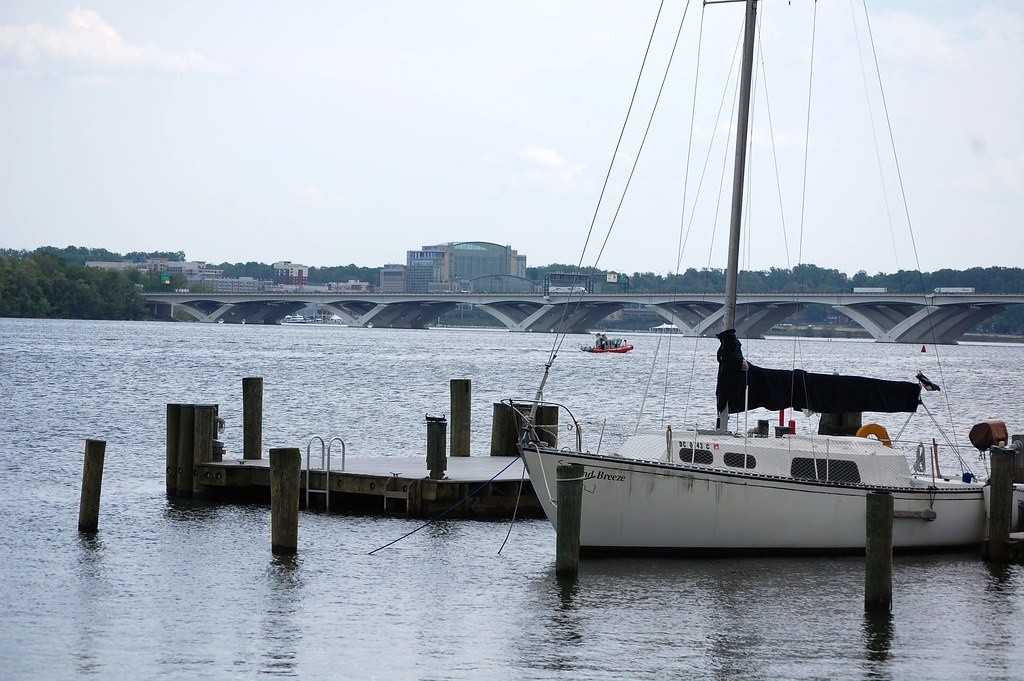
[284,313,308,324]
[580,336,635,354]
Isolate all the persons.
[921,345,926,352]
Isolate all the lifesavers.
[855,424,892,448]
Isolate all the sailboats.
[501,0,1024,563]
